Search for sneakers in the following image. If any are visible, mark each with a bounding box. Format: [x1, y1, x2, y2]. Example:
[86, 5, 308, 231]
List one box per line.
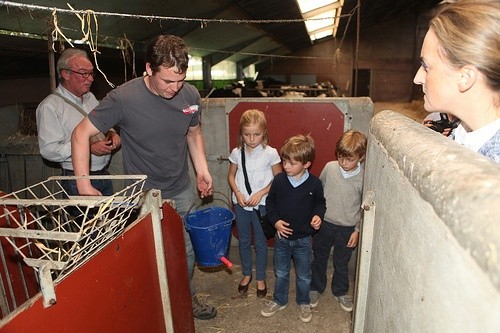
[261, 300, 288, 317]
[333, 294, 353, 312]
[298, 304, 312, 323]
[193, 305, 217, 319]
[308, 290, 321, 307]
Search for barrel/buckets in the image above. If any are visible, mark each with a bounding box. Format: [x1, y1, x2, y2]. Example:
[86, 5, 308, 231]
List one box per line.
[183, 190, 236, 268]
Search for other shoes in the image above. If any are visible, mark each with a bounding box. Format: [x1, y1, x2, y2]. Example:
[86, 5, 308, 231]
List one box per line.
[239, 274, 252, 294]
[257, 280, 266, 298]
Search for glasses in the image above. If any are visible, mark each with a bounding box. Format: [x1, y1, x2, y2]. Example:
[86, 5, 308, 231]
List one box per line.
[60, 69, 97, 78]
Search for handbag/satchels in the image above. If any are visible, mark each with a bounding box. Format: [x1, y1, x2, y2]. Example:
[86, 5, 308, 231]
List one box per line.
[259, 215, 275, 239]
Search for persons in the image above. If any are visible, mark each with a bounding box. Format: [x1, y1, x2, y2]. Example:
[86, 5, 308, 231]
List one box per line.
[413, 0, 500, 162]
[36, 49, 121, 260]
[71, 34, 217, 320]
[260, 132, 327, 322]
[228, 109, 282, 298]
[309, 129, 368, 312]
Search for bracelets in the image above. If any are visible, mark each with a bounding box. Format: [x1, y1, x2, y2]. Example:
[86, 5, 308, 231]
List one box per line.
[235, 190, 240, 194]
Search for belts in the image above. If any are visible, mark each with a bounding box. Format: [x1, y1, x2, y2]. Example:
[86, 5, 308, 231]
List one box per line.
[61, 169, 100, 175]
